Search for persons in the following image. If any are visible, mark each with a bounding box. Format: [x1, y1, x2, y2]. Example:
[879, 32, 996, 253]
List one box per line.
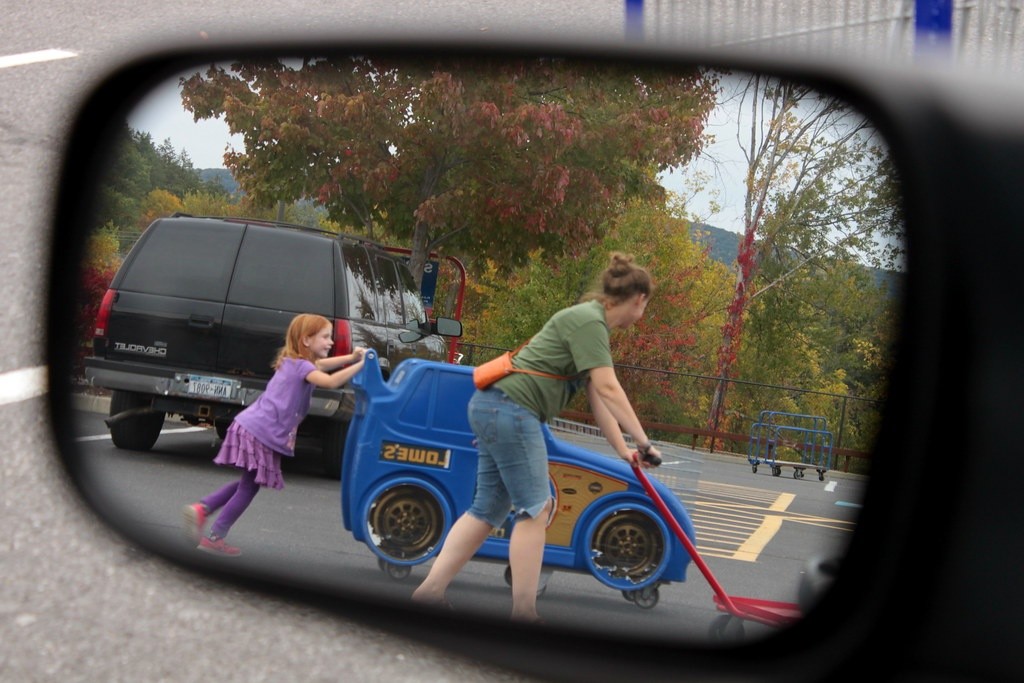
[410, 252, 662, 626]
[182, 315, 367, 557]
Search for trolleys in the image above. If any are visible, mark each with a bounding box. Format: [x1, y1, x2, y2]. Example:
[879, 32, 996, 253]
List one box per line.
[746, 410, 834, 481]
[628, 448, 810, 643]
[338, 348, 698, 612]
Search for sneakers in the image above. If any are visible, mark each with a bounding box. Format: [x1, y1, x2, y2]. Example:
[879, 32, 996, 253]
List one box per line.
[196, 536, 240, 557]
[185, 503, 206, 547]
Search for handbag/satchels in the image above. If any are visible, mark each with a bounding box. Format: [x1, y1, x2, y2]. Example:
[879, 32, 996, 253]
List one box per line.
[473, 351, 515, 389]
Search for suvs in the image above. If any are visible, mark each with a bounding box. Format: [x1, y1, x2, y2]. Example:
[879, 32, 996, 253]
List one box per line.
[82, 211, 463, 482]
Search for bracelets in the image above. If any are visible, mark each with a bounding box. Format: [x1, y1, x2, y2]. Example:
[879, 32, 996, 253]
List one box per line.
[637, 444, 651, 454]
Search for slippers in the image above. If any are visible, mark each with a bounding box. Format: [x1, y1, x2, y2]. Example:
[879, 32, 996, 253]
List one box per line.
[408, 595, 455, 611]
[511, 613, 549, 626]
